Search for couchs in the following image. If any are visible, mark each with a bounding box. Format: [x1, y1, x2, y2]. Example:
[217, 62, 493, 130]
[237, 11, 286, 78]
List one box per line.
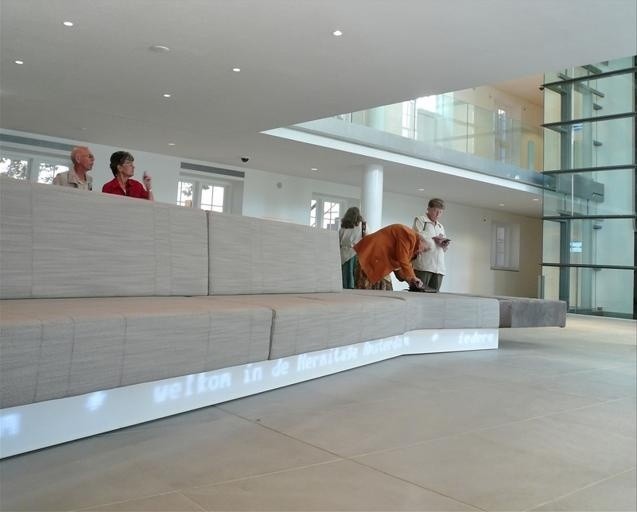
[0, 174, 499, 460]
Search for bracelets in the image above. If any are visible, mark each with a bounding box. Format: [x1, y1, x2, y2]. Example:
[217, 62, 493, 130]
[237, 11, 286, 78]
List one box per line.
[149, 188, 153, 192]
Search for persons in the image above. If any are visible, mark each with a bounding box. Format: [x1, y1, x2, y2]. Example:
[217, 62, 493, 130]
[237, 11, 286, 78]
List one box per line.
[353, 224, 434, 291]
[410, 198, 449, 293]
[339, 207, 370, 289]
[102, 151, 154, 201]
[52, 146, 94, 191]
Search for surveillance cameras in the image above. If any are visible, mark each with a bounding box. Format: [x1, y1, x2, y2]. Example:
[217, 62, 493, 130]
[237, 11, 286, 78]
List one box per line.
[241, 157, 249, 163]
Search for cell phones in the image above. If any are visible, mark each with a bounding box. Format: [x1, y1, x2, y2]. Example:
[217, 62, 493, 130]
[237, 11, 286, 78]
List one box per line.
[443, 239, 450, 242]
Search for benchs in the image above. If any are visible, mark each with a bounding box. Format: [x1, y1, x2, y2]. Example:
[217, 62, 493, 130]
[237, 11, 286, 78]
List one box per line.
[499, 296, 567, 328]
